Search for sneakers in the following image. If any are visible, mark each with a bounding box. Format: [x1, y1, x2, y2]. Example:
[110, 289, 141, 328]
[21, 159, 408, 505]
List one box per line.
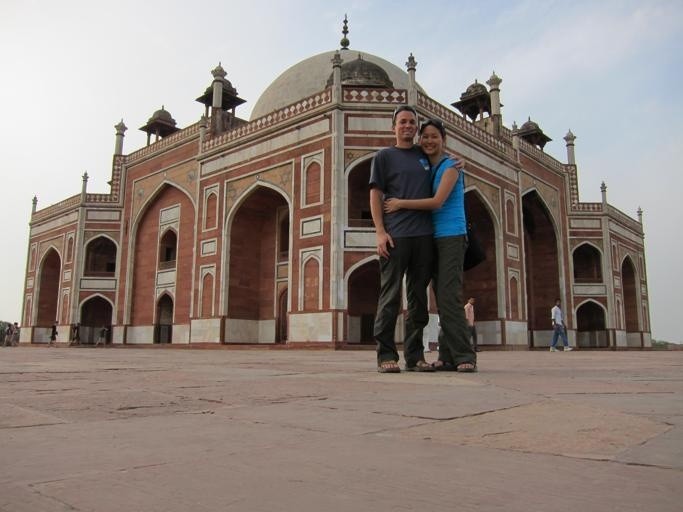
[563, 348, 572, 352]
[550, 346, 558, 352]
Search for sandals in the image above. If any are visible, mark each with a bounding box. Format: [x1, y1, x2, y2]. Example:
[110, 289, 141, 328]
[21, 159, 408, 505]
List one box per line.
[377, 361, 400, 372]
[405, 362, 475, 372]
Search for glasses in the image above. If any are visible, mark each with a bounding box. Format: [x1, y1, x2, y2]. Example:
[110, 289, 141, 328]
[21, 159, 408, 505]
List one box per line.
[393, 106, 416, 117]
[421, 119, 444, 132]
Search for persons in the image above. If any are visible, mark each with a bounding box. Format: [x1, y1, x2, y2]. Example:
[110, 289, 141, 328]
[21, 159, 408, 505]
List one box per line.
[3, 322, 109, 347]
[369, 106, 481, 373]
[549, 298, 573, 352]
